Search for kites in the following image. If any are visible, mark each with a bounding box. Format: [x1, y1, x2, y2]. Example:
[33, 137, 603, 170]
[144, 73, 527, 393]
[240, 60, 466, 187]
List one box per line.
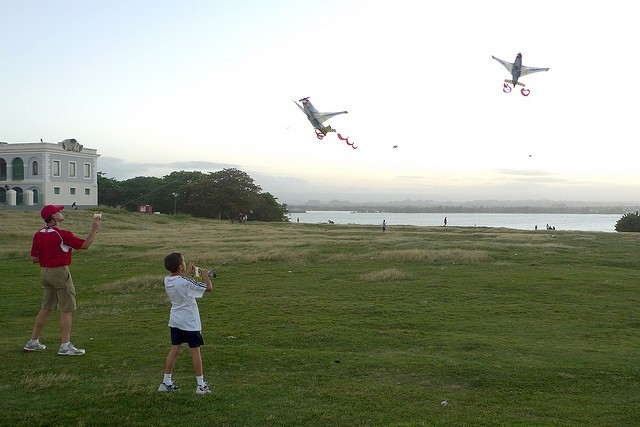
[293, 96, 358, 150]
[529, 155, 532, 157]
[393, 144, 398, 148]
[491, 52, 549, 96]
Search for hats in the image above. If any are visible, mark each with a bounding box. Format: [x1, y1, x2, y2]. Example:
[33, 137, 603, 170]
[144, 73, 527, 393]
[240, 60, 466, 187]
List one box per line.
[41, 205, 64, 219]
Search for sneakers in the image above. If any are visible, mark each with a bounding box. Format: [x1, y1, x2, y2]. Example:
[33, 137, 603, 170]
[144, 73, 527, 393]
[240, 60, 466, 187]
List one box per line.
[58, 343, 85, 355]
[158, 383, 179, 391]
[24, 341, 46, 351]
[196, 384, 211, 394]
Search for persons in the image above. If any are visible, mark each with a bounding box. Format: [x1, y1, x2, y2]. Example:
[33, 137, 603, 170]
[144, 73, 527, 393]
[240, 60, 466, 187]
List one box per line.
[382, 219, 387, 232]
[297, 217, 300, 223]
[24, 204, 102, 356]
[239, 212, 247, 224]
[158, 252, 212, 394]
[444, 217, 447, 226]
[546, 223, 555, 230]
[534, 224, 538, 229]
